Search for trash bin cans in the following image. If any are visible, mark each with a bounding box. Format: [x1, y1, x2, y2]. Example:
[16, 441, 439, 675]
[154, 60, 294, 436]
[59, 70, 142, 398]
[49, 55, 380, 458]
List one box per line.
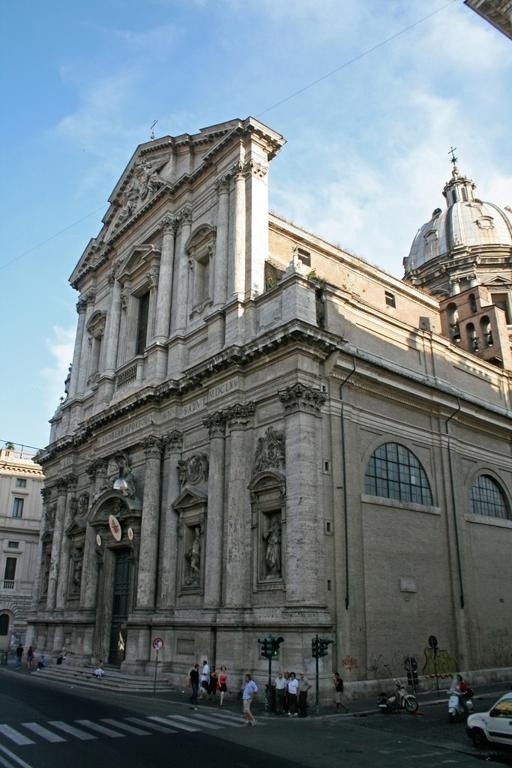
[0, 651, 8, 665]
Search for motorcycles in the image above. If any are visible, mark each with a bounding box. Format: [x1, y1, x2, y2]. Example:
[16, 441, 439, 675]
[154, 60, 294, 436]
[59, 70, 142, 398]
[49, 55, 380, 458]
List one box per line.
[446, 680, 474, 720]
[376, 678, 419, 714]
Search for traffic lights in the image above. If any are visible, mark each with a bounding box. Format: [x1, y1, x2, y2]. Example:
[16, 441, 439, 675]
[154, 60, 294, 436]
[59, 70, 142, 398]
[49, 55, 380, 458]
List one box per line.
[271, 637, 284, 657]
[263, 638, 273, 658]
[312, 638, 320, 658]
[258, 638, 265, 657]
[320, 639, 329, 657]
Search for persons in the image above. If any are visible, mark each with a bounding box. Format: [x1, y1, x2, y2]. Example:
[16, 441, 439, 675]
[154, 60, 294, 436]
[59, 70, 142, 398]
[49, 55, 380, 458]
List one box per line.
[14, 643, 24, 670]
[206, 666, 217, 707]
[332, 672, 350, 713]
[242, 674, 258, 728]
[450, 673, 468, 716]
[260, 520, 281, 577]
[188, 663, 199, 704]
[274, 671, 299, 718]
[94, 659, 105, 679]
[26, 645, 35, 670]
[216, 665, 228, 709]
[298, 672, 312, 718]
[180, 527, 201, 586]
[197, 660, 210, 702]
[37, 655, 49, 671]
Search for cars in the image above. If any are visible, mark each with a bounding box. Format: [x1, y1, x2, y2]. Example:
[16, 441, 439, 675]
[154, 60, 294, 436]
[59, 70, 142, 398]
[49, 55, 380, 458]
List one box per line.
[466, 691, 512, 749]
